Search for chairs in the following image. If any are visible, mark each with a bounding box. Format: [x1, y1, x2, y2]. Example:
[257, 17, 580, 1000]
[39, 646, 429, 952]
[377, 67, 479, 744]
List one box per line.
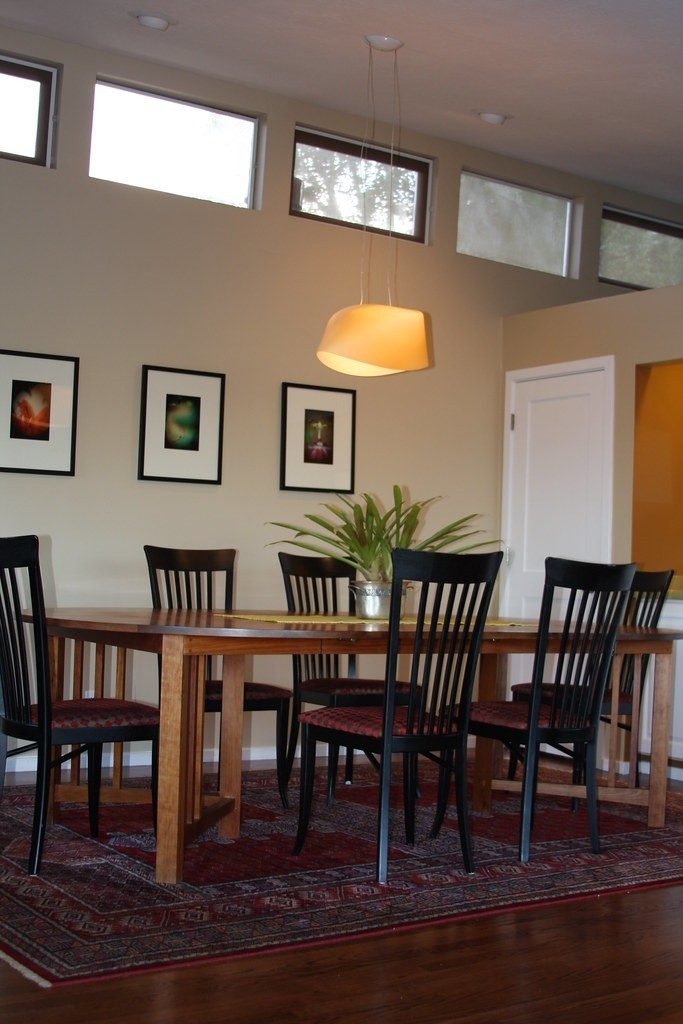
[428, 557, 637, 865]
[279, 552, 422, 806]
[0, 534, 160, 876]
[144, 544, 291, 809]
[506, 568, 675, 812]
[292, 548, 504, 885]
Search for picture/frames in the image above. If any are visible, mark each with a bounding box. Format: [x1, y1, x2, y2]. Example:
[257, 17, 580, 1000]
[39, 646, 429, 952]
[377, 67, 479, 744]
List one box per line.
[0, 349, 80, 477]
[137, 365, 225, 485]
[280, 381, 357, 494]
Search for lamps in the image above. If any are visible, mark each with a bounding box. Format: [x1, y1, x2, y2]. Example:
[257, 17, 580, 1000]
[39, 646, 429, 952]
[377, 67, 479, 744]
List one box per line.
[317, 35, 430, 377]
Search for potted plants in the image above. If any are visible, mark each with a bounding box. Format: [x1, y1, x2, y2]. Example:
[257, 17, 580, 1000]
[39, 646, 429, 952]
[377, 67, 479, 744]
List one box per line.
[264, 486, 506, 619]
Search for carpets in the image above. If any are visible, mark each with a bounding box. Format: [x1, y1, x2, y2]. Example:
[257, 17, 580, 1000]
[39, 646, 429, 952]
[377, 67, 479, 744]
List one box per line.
[0, 764, 683, 993]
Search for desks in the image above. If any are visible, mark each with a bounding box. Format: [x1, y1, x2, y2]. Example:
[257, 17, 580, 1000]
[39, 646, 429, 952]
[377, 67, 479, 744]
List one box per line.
[14, 604, 683, 885]
